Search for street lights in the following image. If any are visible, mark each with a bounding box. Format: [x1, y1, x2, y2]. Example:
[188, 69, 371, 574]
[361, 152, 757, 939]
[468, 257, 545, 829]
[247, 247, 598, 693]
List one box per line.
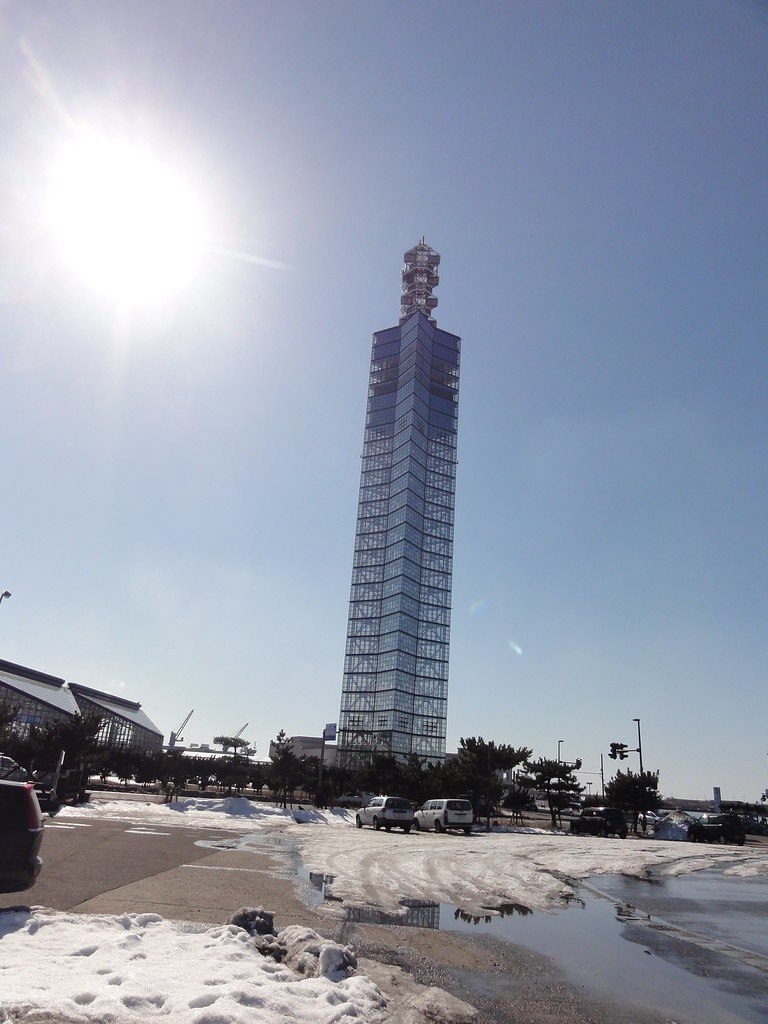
[632, 719, 643, 775]
[557, 740, 567, 811]
[587, 782, 593, 801]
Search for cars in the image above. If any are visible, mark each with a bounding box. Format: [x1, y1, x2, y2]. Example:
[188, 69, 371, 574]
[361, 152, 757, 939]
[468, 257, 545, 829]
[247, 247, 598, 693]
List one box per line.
[0, 751, 29, 783]
[0, 778, 48, 893]
[638, 809, 668, 826]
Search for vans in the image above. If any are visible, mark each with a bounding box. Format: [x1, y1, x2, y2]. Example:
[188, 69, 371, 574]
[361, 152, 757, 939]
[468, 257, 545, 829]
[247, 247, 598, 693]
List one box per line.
[414, 798, 474, 835]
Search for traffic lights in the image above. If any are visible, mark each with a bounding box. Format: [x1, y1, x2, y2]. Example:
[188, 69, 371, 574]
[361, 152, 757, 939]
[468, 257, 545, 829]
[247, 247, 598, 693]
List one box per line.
[620, 742, 628, 760]
[607, 743, 617, 759]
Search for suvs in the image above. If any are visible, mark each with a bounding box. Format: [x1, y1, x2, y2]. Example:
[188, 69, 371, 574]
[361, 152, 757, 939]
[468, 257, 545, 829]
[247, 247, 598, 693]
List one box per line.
[570, 806, 628, 840]
[688, 812, 745, 846]
[355, 796, 415, 834]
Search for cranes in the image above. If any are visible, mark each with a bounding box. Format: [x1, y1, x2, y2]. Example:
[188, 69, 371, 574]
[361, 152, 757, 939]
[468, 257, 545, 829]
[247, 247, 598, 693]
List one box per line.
[222, 722, 250, 752]
[168, 709, 195, 748]
